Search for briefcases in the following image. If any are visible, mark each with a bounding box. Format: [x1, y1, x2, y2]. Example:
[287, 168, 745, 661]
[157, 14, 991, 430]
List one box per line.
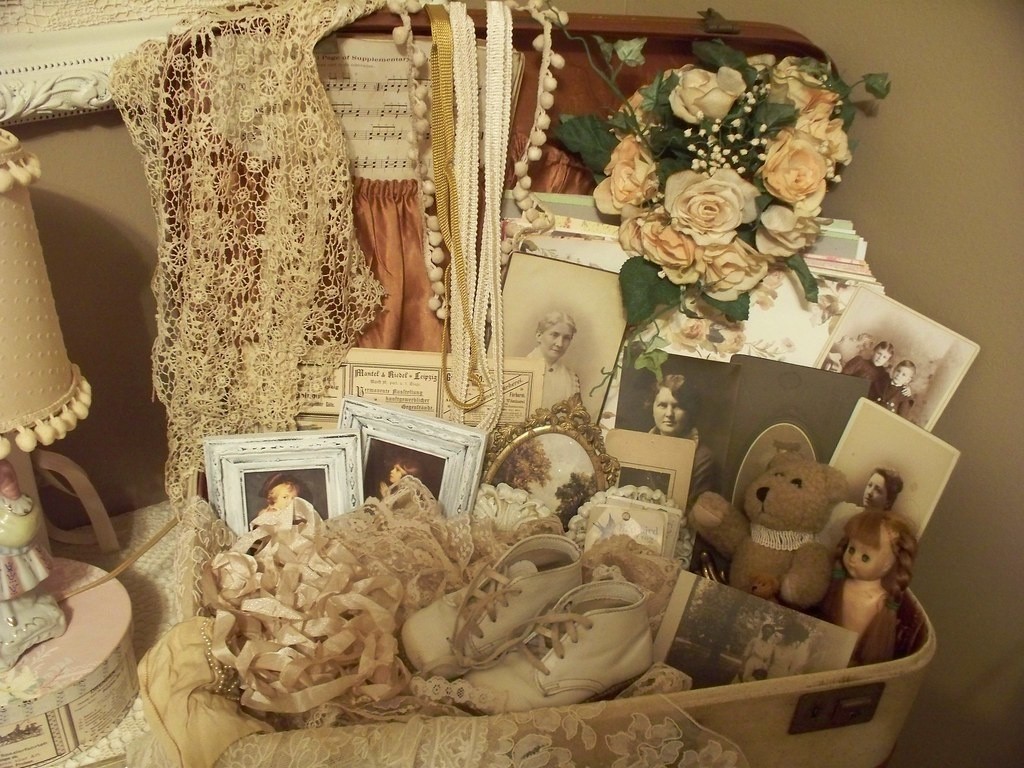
[157, 6, 938, 768]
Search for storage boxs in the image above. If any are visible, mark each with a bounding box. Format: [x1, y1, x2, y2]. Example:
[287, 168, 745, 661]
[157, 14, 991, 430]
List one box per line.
[138, 0, 938, 768]
[0, 558, 140, 768]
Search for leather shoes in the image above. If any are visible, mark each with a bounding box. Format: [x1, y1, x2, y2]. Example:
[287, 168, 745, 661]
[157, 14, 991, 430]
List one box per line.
[398, 534, 583, 679]
[462, 580, 653, 716]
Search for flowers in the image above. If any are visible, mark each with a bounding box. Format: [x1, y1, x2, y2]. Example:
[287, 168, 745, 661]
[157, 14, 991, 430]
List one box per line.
[540, 10, 896, 431]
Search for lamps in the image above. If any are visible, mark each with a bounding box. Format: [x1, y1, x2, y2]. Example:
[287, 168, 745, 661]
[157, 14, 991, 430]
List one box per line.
[0, 128, 135, 558]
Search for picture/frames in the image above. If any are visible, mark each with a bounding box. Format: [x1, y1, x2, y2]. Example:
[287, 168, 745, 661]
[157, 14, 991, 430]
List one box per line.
[485, 251, 630, 423]
[339, 392, 489, 520]
[811, 283, 981, 432]
[829, 397, 961, 543]
[202, 428, 365, 559]
[565, 483, 693, 575]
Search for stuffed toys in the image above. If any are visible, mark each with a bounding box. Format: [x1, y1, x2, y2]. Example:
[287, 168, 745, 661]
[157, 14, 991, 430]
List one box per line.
[688, 454, 849, 610]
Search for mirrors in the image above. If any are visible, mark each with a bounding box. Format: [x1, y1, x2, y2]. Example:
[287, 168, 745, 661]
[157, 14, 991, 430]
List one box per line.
[478, 392, 621, 532]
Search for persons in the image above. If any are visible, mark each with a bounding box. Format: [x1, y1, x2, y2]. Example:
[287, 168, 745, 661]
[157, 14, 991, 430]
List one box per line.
[379, 456, 424, 500]
[819, 466, 904, 549]
[824, 333, 916, 419]
[813, 507, 916, 722]
[639, 374, 721, 508]
[259, 472, 309, 507]
[731, 623, 777, 683]
[524, 312, 581, 409]
